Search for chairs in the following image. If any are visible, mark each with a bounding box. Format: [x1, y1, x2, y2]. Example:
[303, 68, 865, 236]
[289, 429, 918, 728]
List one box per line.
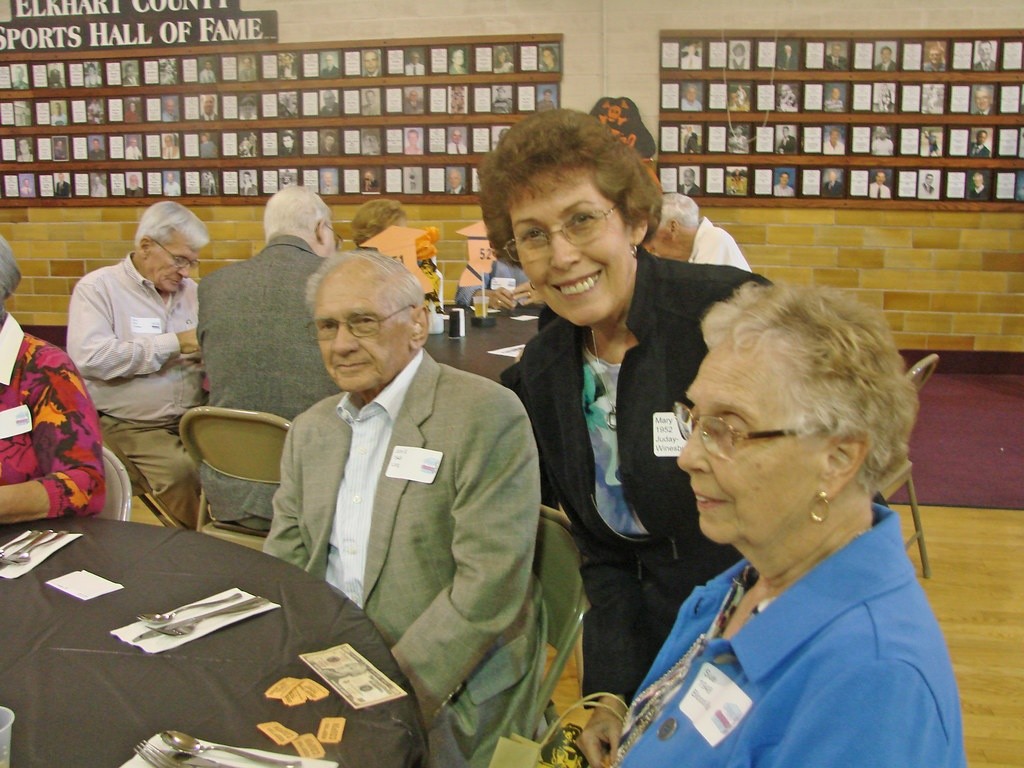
[178, 406, 292, 554]
[532, 503, 589, 728]
[877, 354, 940, 577]
[96, 445, 131, 522]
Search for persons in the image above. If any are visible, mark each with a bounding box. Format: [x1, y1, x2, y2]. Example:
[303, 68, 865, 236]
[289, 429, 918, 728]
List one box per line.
[675, 37, 996, 203]
[644, 194, 752, 272]
[478, 108, 778, 768]
[198, 184, 342, 527]
[10, 44, 559, 203]
[609, 279, 964, 768]
[455, 254, 546, 310]
[264, 242, 541, 768]
[351, 199, 405, 251]
[66, 200, 210, 530]
[0, 232, 106, 525]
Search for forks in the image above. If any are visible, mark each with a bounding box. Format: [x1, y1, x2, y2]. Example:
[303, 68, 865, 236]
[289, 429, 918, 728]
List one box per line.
[133, 739, 205, 768]
[1, 530, 70, 564]
[146, 597, 269, 637]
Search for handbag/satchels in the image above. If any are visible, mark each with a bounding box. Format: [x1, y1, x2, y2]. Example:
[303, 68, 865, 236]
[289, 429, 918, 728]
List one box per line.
[490, 693, 629, 768]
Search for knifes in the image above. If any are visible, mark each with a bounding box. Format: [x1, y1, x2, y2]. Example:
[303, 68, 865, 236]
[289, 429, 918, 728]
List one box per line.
[160, 749, 235, 768]
[0, 529, 53, 565]
[132, 596, 262, 643]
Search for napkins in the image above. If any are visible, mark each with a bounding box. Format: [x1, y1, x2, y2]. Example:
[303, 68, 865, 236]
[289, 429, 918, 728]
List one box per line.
[109, 586, 282, 653]
[0, 530, 83, 580]
[47, 570, 124, 600]
[119, 734, 339, 768]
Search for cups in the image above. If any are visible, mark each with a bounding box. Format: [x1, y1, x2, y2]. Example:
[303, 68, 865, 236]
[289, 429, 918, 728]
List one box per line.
[472, 296, 489, 318]
[0, 705, 16, 768]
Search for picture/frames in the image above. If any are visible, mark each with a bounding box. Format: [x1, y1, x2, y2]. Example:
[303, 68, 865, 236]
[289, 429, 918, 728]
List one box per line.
[655, 38, 1023, 204]
[9, 40, 564, 199]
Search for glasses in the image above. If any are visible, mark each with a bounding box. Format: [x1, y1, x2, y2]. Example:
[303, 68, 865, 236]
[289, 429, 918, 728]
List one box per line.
[318, 221, 343, 250]
[146, 235, 201, 269]
[306, 305, 415, 341]
[504, 203, 620, 263]
[673, 402, 837, 458]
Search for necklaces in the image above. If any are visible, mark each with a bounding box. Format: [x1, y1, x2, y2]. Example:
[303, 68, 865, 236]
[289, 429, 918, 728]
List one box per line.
[606, 523, 874, 768]
[590, 328, 616, 430]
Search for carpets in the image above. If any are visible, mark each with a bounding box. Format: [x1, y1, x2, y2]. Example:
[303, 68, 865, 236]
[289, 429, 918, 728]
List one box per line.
[886, 373, 1024, 511]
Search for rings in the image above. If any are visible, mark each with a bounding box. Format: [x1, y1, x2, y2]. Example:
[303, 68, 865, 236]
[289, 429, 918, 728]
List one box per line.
[527, 292, 531, 296]
[498, 300, 501, 303]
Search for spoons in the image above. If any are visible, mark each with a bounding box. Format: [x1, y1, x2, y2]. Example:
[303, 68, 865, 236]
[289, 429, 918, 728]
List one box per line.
[161, 730, 302, 768]
[0, 529, 41, 558]
[136, 593, 243, 625]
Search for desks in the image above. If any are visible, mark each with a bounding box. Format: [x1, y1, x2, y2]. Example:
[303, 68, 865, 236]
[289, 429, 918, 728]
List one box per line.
[419, 304, 542, 383]
[0, 515, 435, 768]
